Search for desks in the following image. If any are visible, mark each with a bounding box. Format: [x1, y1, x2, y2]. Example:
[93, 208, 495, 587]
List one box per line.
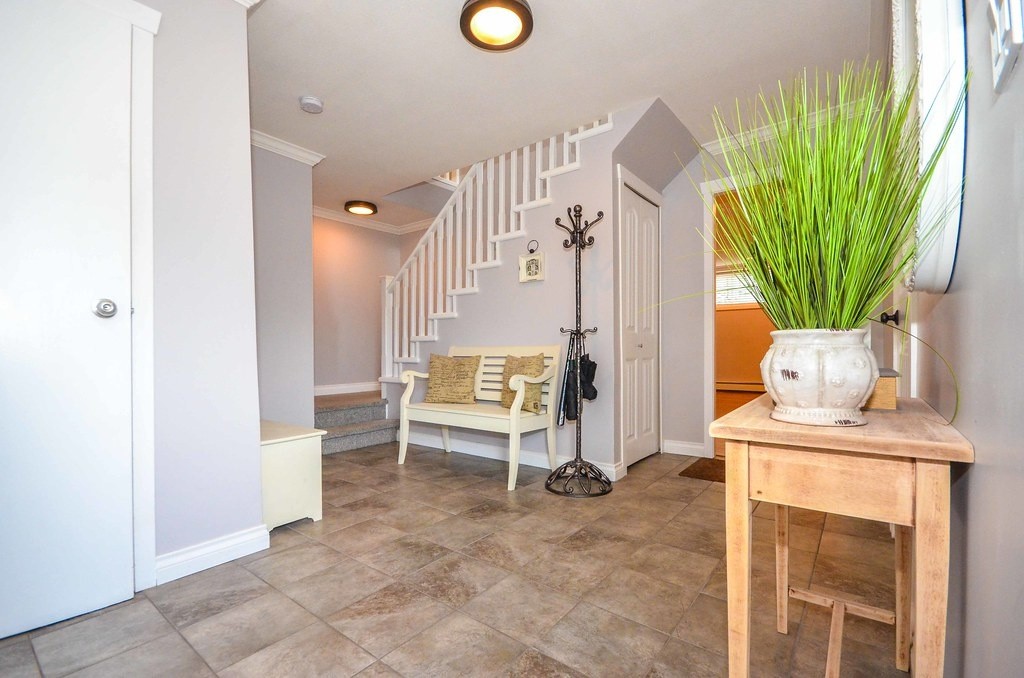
[707, 391, 975, 678]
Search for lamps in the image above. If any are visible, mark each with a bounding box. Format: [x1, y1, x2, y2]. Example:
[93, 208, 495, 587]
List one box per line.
[459, 0, 533, 51]
[343, 199, 377, 216]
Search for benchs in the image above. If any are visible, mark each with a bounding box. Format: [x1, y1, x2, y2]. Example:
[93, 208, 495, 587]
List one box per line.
[396, 345, 565, 491]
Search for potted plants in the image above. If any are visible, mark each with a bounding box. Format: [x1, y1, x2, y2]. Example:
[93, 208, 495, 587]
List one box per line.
[675, 54, 973, 429]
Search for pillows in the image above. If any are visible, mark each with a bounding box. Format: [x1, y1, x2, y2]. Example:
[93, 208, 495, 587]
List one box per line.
[501, 352, 545, 414]
[423, 353, 481, 404]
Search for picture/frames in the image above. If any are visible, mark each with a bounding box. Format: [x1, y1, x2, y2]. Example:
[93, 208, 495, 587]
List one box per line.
[518, 251, 544, 282]
[889, 0, 968, 294]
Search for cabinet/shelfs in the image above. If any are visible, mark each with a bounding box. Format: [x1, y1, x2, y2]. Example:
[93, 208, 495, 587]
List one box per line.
[259, 417, 327, 534]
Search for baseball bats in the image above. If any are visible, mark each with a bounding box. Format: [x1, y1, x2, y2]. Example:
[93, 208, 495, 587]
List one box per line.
[557, 330, 575, 426]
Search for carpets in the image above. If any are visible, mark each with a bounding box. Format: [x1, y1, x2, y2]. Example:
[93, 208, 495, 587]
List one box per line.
[678, 457, 725, 483]
